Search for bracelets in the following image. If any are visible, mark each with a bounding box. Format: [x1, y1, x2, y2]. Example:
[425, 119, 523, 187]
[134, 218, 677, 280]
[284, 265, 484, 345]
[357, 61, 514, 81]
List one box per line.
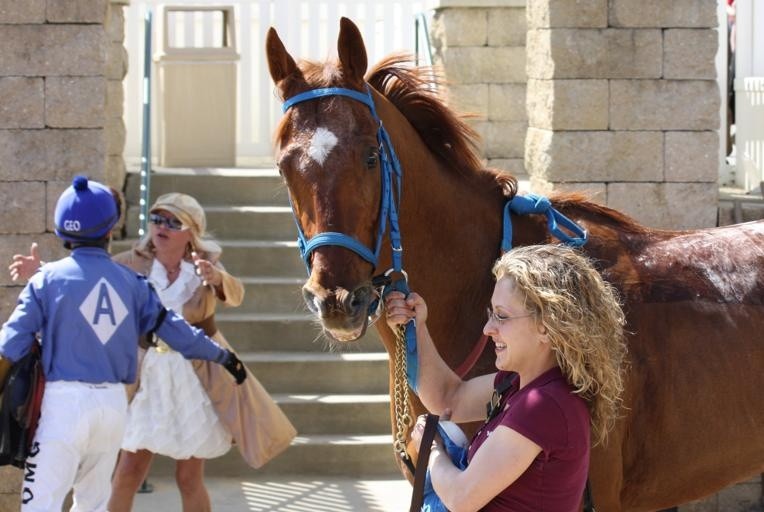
[430, 441, 442, 449]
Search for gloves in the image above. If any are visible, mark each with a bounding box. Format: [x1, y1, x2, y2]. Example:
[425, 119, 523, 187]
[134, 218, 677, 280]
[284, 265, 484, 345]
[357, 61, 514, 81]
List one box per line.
[224, 349, 246, 383]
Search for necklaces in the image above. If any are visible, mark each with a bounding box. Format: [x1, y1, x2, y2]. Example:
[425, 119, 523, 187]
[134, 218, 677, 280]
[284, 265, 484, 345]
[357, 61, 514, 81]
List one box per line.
[167, 268, 178, 273]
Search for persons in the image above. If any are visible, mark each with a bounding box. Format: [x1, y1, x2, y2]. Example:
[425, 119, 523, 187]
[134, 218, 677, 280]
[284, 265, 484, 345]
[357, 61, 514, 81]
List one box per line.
[8, 191, 298, 512]
[385, 244, 632, 511]
[1, 176, 247, 511]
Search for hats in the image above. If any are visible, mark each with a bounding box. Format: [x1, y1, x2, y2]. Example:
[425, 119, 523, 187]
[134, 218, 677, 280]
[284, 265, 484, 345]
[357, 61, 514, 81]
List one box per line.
[54, 177, 121, 242]
[148, 193, 205, 233]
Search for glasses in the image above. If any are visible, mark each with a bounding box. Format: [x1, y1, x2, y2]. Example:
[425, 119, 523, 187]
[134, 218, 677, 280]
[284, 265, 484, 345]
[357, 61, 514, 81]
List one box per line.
[486, 307, 533, 322]
[150, 214, 184, 229]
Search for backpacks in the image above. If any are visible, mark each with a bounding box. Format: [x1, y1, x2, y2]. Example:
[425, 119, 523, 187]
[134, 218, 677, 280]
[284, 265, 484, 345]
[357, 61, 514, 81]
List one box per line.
[0, 340, 45, 467]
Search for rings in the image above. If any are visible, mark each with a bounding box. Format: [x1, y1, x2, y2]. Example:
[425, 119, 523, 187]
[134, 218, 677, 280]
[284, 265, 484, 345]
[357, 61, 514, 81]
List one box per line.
[388, 311, 391, 316]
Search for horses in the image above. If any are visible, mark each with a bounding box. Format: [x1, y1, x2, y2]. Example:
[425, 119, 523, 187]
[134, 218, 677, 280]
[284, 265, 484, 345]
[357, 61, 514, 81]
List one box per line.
[262, 15, 764, 512]
